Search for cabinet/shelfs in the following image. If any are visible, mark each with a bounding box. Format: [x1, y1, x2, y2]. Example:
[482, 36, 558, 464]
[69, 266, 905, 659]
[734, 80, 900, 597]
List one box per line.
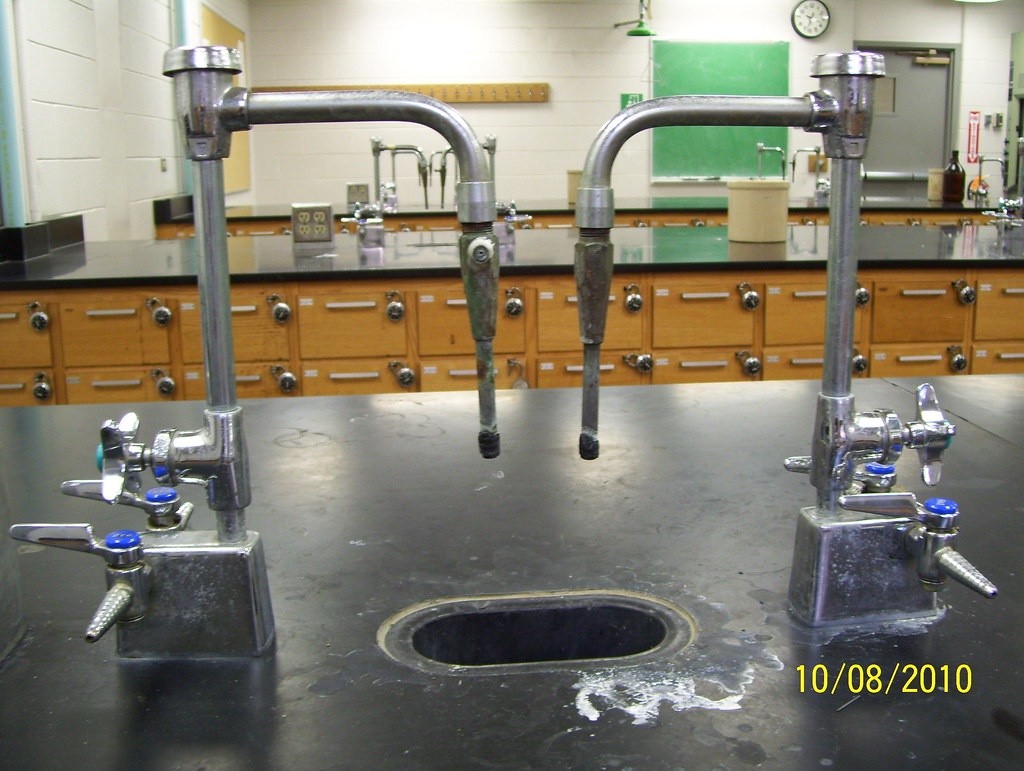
[1, 268, 1022, 404]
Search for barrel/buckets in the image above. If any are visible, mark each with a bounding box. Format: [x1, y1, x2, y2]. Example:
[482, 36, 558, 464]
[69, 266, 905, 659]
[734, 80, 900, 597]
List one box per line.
[726, 178, 791, 245]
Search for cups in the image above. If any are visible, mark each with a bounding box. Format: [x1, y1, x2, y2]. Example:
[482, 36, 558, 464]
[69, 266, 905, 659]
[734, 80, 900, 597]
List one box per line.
[928, 169, 943, 201]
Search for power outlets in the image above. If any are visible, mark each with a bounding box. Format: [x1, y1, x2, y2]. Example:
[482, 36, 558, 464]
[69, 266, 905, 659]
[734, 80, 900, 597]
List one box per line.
[346, 183, 369, 204]
[292, 204, 334, 244]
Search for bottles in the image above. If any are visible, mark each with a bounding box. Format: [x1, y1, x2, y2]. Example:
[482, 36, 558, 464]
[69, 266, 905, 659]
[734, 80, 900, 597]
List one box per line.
[942, 151, 965, 203]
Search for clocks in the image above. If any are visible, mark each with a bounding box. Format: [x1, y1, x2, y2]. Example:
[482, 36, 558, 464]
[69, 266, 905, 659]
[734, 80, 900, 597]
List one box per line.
[791, 0, 830, 39]
